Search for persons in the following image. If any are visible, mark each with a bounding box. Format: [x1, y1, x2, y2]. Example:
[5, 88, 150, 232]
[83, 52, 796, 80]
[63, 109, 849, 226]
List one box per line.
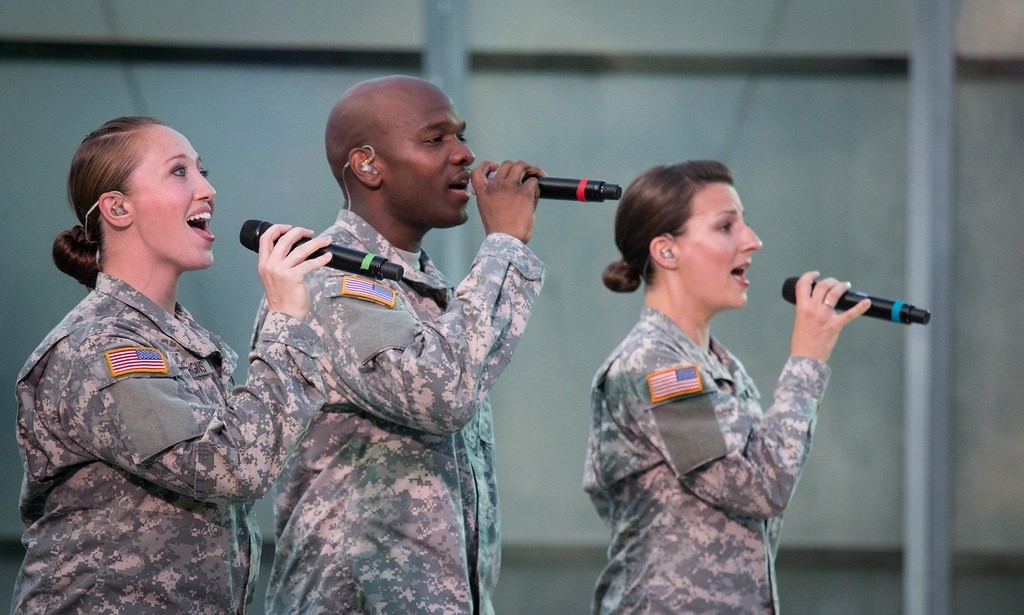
[583, 159, 871, 615]
[251, 75, 546, 615]
[12, 116, 332, 615]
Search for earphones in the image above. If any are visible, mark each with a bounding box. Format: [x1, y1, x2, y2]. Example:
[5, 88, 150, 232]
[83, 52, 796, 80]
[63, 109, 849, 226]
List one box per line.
[660, 247, 674, 258]
[361, 159, 377, 175]
[112, 199, 126, 215]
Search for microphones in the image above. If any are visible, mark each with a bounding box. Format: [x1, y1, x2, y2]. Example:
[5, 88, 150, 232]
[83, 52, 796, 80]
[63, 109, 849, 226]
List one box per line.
[239, 220, 404, 282]
[469, 172, 622, 201]
[782, 278, 932, 325]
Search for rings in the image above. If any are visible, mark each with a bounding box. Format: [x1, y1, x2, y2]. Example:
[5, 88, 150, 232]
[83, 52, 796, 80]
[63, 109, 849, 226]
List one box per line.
[824, 300, 834, 308]
